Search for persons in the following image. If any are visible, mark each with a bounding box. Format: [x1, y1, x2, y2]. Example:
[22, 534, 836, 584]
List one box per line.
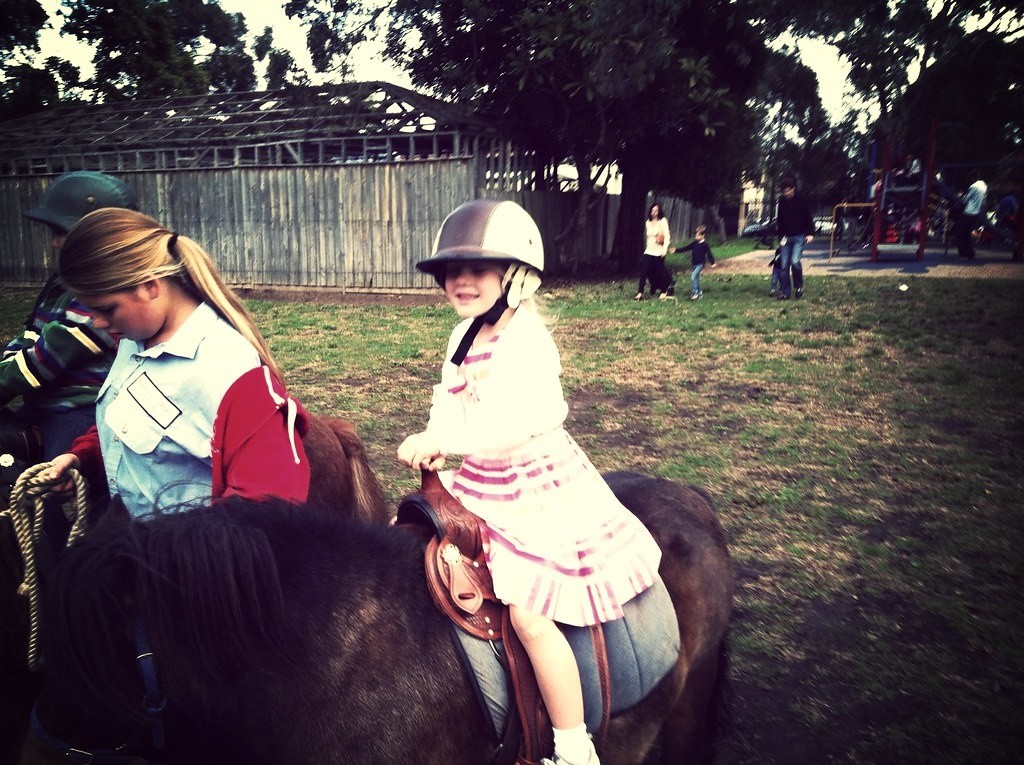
[945, 170, 990, 263]
[668, 224, 717, 301]
[398, 197, 662, 765]
[895, 150, 924, 216]
[994, 190, 1018, 246]
[634, 203, 673, 300]
[34, 207, 314, 531]
[0, 171, 142, 540]
[769, 178, 816, 299]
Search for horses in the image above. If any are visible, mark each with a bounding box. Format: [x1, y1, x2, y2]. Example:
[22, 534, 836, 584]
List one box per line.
[1, 416, 734, 765]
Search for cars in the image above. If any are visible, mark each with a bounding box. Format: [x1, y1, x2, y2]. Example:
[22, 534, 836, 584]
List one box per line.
[741, 217, 779, 237]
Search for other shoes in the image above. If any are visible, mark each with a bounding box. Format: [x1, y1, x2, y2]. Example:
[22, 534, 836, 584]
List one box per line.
[666, 287, 675, 299]
[698, 295, 704, 300]
[795, 287, 803, 300]
[953, 253, 969, 262]
[645, 294, 655, 300]
[634, 292, 643, 301]
[768, 289, 775, 297]
[691, 294, 698, 299]
[777, 293, 790, 300]
[659, 291, 667, 300]
[541, 733, 600, 765]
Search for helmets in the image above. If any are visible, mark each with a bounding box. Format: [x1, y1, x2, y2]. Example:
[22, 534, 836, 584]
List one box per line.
[416, 200, 544, 273]
[22, 170, 138, 232]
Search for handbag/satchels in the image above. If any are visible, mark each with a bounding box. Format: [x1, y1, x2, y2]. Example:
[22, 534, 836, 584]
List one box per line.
[655, 231, 663, 244]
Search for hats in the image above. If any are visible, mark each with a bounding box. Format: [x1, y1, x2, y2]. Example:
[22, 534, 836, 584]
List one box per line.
[779, 178, 795, 192]
